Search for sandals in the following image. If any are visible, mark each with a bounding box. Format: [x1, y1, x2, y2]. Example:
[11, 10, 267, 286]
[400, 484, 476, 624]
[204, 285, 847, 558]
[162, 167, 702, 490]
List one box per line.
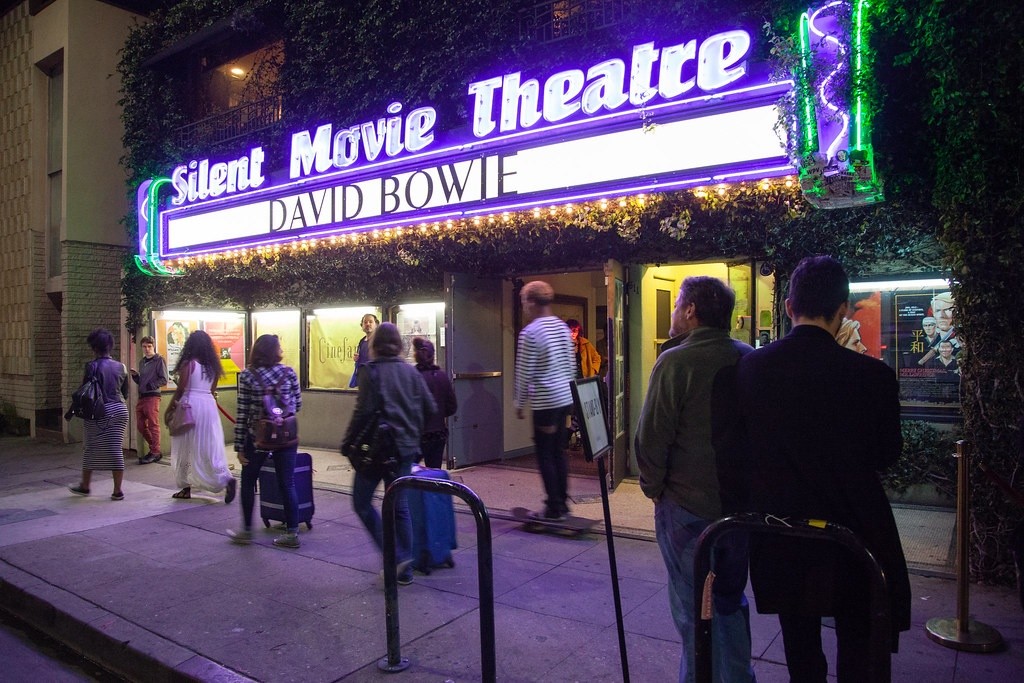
[172, 487, 191, 498]
[225, 478, 236, 503]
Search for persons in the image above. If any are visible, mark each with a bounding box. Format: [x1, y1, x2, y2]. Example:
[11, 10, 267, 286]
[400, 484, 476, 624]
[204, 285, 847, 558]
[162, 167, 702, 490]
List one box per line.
[225, 335, 301, 547]
[835, 319, 866, 354]
[711, 254, 912, 683]
[340, 322, 437, 585]
[63, 328, 129, 499]
[636, 277, 759, 683]
[350, 314, 380, 388]
[133, 336, 166, 460]
[411, 337, 457, 468]
[514, 281, 575, 522]
[566, 319, 601, 450]
[161, 331, 236, 502]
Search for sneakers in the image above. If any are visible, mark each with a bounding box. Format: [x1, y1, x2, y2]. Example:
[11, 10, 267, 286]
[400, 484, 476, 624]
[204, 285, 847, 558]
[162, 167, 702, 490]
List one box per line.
[111, 492, 124, 500]
[68, 484, 90, 496]
[224, 528, 253, 544]
[272, 534, 302, 548]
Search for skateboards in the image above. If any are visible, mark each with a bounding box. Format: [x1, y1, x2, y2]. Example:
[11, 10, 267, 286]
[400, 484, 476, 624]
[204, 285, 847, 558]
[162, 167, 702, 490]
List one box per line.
[513, 506, 604, 539]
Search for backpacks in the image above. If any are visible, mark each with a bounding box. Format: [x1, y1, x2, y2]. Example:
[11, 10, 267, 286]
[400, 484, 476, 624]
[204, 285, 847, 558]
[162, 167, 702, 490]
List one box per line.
[249, 367, 299, 450]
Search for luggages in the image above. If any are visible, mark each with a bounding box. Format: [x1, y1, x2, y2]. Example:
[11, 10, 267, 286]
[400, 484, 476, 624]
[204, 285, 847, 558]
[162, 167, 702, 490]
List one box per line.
[407, 463, 458, 575]
[259, 453, 315, 530]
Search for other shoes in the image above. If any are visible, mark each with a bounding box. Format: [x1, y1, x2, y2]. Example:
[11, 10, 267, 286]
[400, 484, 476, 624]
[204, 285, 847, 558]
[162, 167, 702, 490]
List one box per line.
[527, 511, 567, 521]
[138, 451, 162, 464]
[379, 559, 415, 586]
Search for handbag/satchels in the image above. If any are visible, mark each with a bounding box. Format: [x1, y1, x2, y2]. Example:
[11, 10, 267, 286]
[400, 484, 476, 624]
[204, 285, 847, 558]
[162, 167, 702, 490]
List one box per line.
[165, 403, 195, 436]
[349, 362, 359, 388]
[71, 362, 106, 420]
[348, 422, 404, 481]
